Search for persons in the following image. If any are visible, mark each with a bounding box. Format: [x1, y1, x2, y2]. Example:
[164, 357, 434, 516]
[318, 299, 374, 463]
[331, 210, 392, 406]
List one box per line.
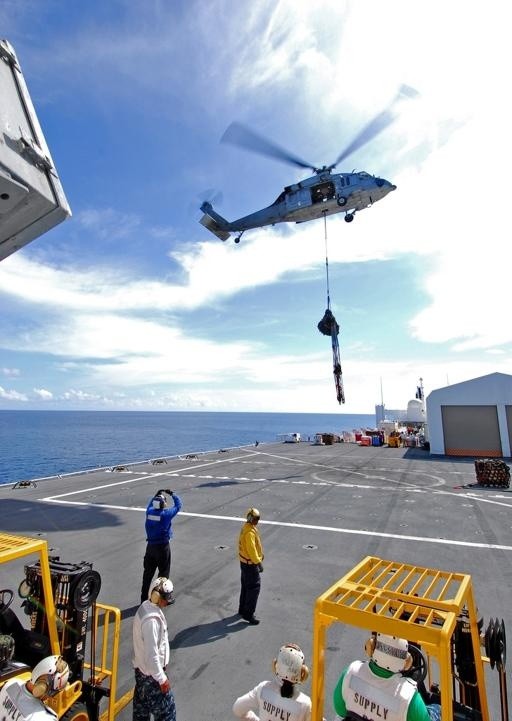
[0, 634, 30, 677]
[1, 655, 70, 721]
[333, 632, 442, 720]
[398, 425, 418, 448]
[140, 489, 184, 604]
[130, 577, 179, 720]
[238, 507, 263, 624]
[233, 643, 312, 721]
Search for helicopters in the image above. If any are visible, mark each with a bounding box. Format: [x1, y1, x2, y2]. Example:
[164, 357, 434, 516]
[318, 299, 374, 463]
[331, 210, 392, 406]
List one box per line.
[199, 85, 421, 244]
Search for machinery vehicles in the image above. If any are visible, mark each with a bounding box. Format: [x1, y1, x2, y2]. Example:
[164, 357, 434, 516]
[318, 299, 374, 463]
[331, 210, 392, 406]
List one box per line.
[1, 532, 137, 721]
[386, 429, 400, 449]
[310, 552, 508, 721]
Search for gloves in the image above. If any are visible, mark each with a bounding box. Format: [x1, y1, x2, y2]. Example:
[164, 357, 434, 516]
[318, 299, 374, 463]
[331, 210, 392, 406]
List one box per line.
[165, 490, 174, 496]
[160, 678, 171, 694]
[155, 490, 164, 495]
[259, 564, 263, 573]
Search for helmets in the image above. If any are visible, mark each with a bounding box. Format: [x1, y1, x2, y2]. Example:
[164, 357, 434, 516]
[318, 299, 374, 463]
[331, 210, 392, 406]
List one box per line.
[149, 577, 175, 607]
[152, 494, 168, 509]
[30, 655, 70, 699]
[272, 643, 309, 684]
[364, 633, 413, 673]
[246, 508, 260, 523]
